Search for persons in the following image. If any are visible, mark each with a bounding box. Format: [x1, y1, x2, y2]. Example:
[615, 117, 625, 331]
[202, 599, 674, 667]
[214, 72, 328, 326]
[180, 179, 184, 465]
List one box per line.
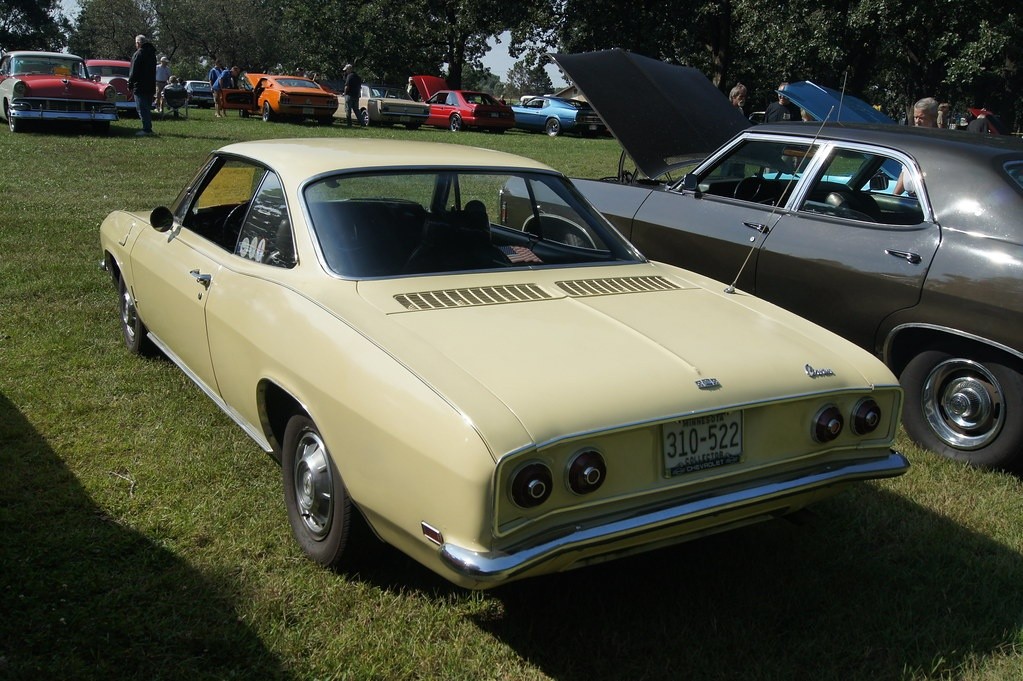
[499, 95, 506, 105]
[128, 34, 157, 135]
[156, 56, 187, 116]
[296, 67, 316, 81]
[343, 64, 366, 128]
[209, 59, 250, 118]
[405, 77, 418, 101]
[893, 97, 939, 196]
[967, 114, 988, 134]
[937, 104, 949, 129]
[760, 82, 816, 173]
[721, 83, 747, 177]
[956, 108, 973, 131]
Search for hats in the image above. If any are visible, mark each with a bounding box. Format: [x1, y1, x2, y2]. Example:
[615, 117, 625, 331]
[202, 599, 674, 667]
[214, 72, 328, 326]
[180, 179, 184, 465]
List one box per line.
[342, 63, 352, 72]
[161, 56, 171, 63]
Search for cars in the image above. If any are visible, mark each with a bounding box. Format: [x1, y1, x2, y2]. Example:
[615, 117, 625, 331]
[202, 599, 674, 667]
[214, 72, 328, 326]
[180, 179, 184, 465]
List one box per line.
[216, 74, 338, 125]
[79, 59, 139, 118]
[411, 76, 516, 135]
[100, 137, 912, 592]
[0, 50, 119, 133]
[333, 83, 430, 128]
[511, 96, 607, 137]
[180, 80, 215, 109]
[499, 50, 1023, 465]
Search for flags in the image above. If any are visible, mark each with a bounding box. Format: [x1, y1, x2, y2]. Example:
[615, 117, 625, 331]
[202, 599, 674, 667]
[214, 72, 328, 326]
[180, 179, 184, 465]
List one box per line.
[500, 246, 543, 262]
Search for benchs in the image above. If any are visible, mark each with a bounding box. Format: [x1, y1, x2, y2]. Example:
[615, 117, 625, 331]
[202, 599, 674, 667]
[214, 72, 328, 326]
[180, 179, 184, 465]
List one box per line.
[309, 202, 434, 277]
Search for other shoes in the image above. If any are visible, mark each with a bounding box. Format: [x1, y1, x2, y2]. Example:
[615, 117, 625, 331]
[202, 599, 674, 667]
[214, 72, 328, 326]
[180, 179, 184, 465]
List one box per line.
[214, 111, 221, 117]
[134, 130, 153, 136]
[361, 123, 367, 128]
[346, 124, 352, 128]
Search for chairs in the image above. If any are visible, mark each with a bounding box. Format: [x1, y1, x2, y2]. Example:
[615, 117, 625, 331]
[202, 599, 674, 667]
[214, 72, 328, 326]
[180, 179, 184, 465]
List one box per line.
[295, 81, 306, 86]
[804, 181, 885, 225]
[161, 87, 189, 120]
[402, 208, 514, 274]
